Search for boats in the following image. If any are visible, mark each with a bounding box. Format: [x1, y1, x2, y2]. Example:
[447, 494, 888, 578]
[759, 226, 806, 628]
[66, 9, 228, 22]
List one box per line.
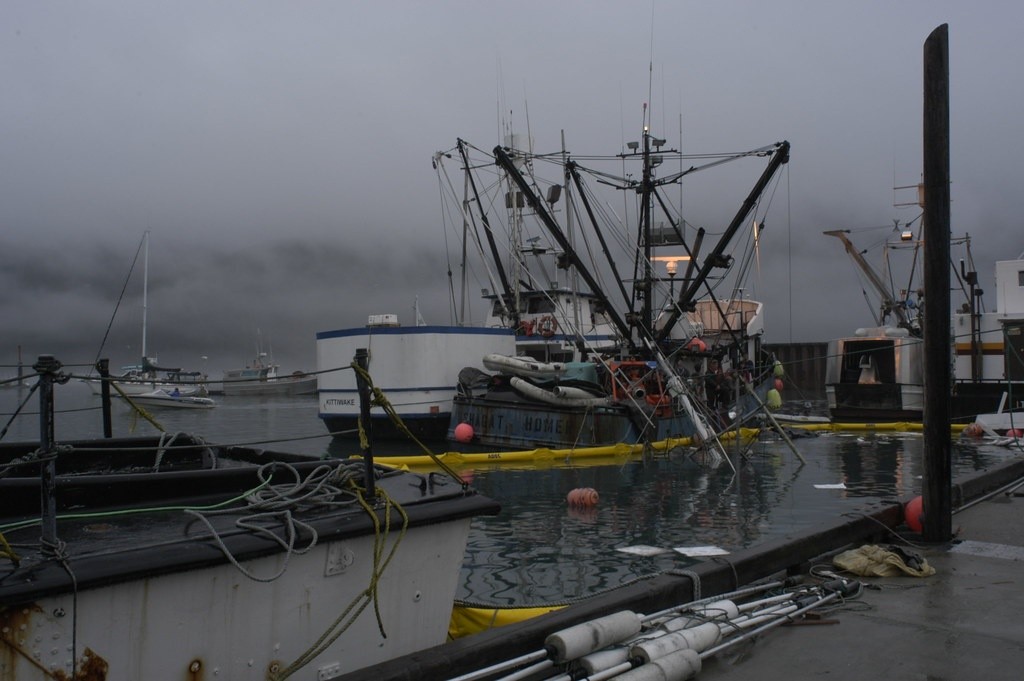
[222, 328, 317, 396]
[314, 60, 1024, 463]
[114, 388, 218, 409]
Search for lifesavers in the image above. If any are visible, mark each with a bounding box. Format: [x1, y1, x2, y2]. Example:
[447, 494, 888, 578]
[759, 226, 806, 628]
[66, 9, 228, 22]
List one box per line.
[538, 315, 557, 337]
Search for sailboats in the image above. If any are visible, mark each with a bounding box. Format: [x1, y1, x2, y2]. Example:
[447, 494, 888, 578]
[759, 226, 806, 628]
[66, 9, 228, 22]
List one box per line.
[74, 228, 210, 397]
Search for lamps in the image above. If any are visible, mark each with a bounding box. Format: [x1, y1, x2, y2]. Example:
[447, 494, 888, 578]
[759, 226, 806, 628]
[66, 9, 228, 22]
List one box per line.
[685, 299, 698, 314]
[481, 288, 489, 296]
[594, 300, 606, 315]
[902, 230, 914, 240]
[550, 282, 558, 290]
[556, 252, 571, 271]
[547, 183, 561, 209]
[652, 140, 666, 151]
[704, 254, 733, 269]
[627, 142, 640, 154]
[624, 311, 640, 325]
